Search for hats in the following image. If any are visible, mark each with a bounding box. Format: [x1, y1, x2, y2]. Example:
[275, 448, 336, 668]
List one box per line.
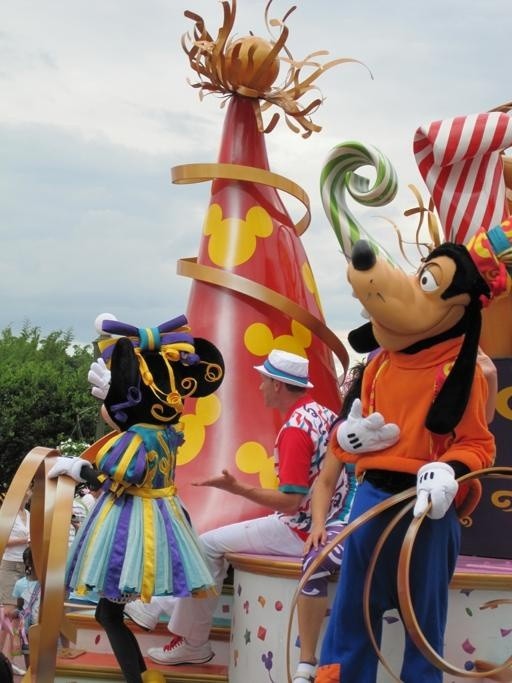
[253, 349, 314, 388]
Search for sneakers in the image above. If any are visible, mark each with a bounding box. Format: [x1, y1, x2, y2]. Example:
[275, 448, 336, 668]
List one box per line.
[147, 635, 215, 665]
[122, 600, 159, 631]
[11, 663, 26, 675]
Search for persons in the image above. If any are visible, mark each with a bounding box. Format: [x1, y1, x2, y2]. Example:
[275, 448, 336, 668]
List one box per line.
[0, 484, 103, 683]
[123, 350, 349, 667]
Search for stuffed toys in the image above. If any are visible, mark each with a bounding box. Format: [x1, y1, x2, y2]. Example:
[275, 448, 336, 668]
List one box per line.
[50, 313, 224, 683]
[313, 216, 512, 683]
